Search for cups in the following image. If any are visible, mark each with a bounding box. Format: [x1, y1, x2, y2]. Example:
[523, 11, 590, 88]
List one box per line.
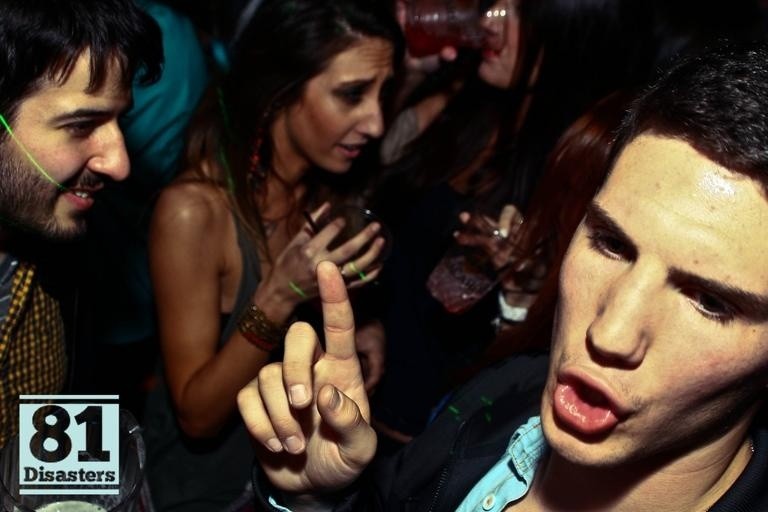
[405, 0, 507, 58]
[329, 204, 393, 277]
[0, 406, 153, 512]
[426, 213, 526, 313]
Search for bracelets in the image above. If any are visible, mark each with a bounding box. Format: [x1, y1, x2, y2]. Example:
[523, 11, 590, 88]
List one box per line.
[236, 301, 282, 352]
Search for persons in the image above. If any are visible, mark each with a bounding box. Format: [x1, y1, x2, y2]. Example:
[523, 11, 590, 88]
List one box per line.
[235, 37, 767, 512]
[0, 0, 166, 450]
[462, 88, 642, 371]
[367, 2, 624, 468]
[150, 1, 403, 438]
